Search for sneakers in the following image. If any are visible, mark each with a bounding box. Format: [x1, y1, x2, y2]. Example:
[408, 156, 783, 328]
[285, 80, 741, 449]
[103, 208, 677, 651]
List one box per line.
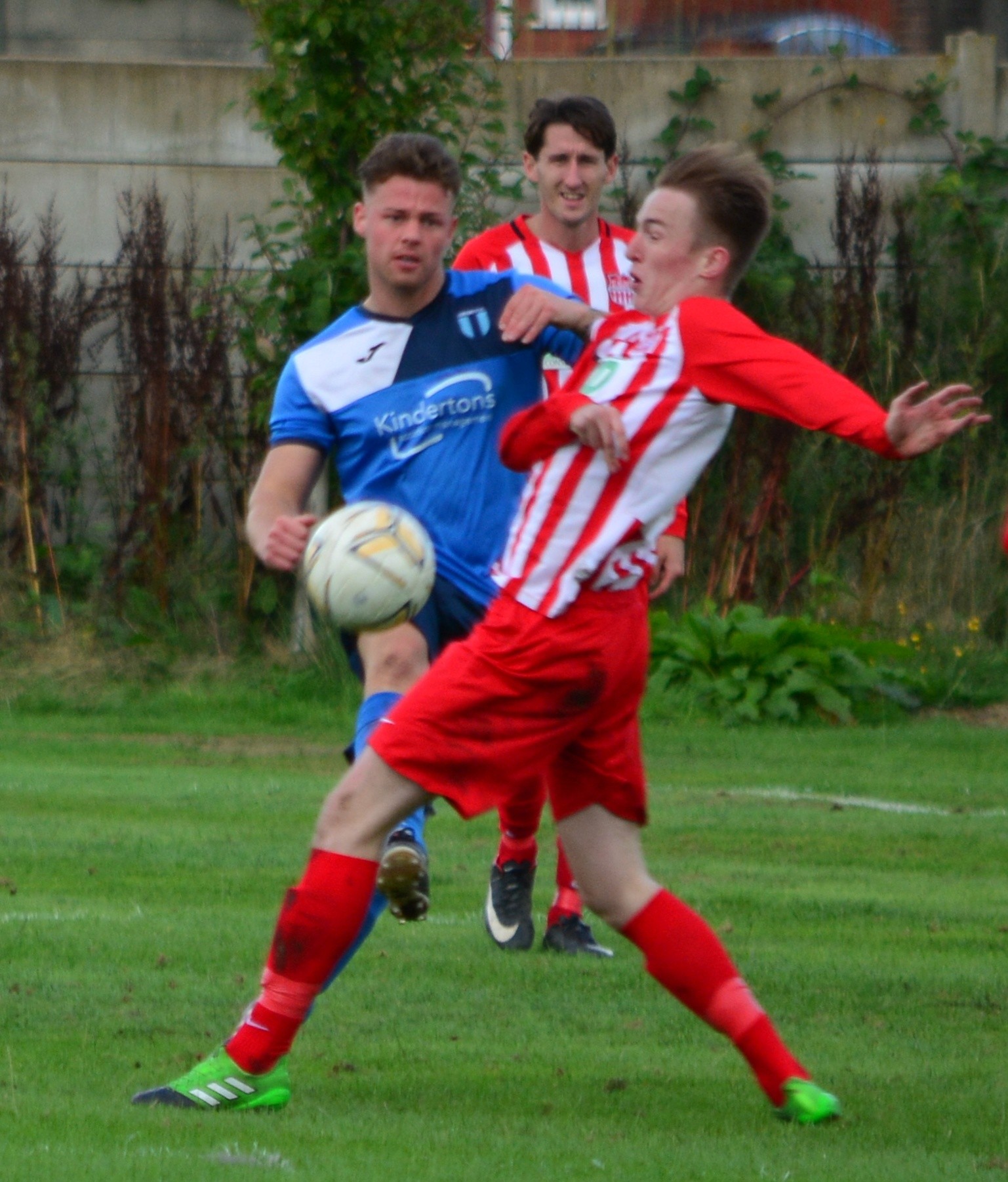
[780, 1079, 841, 1124]
[540, 915, 611, 952]
[130, 1047, 290, 1113]
[485, 855, 535, 949]
[379, 827, 432, 923]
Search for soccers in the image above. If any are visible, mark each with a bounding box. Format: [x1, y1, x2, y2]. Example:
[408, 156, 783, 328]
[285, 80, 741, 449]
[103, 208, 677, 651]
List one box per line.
[303, 501, 437, 634]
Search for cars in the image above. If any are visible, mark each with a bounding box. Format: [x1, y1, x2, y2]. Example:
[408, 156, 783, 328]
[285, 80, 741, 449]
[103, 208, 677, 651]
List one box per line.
[741, 14, 902, 57]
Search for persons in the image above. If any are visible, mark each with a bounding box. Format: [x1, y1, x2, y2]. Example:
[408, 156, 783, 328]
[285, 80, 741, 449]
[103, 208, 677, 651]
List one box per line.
[449, 93, 687, 955]
[244, 136, 612, 1029]
[132, 143, 991, 1113]
